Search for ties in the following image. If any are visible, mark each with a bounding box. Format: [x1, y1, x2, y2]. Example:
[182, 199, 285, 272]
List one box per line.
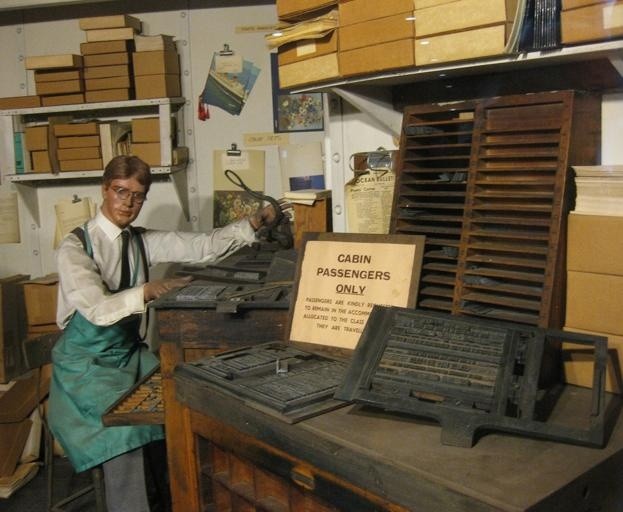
[118, 230, 130, 290]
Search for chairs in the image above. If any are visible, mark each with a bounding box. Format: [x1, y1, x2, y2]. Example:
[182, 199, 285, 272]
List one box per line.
[36, 340, 106, 512]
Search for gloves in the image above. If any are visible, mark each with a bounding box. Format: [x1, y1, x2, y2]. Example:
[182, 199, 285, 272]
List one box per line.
[249, 198, 294, 232]
[144, 276, 192, 302]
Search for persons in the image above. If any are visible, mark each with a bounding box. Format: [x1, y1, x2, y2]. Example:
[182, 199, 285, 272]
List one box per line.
[47, 155, 294, 512]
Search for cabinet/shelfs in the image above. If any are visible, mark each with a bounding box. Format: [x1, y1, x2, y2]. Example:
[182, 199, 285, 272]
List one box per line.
[0, 97, 190, 228]
[289, 37, 623, 140]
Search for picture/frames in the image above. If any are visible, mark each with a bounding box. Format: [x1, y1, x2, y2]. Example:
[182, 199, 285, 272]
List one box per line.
[284, 232, 424, 364]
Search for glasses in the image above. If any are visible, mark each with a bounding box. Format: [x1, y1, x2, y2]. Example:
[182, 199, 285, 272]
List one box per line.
[109, 185, 148, 204]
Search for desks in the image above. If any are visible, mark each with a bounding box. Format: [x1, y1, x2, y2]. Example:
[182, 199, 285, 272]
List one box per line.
[149, 248, 297, 512]
[173, 367, 623, 512]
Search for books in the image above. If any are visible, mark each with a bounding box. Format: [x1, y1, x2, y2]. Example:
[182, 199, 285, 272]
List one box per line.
[570, 165, 623, 216]
[287, 199, 315, 205]
[54, 198, 95, 249]
[135, 34, 176, 51]
[285, 189, 331, 200]
[16, 273, 59, 285]
[506, 0, 562, 53]
[100, 121, 132, 169]
